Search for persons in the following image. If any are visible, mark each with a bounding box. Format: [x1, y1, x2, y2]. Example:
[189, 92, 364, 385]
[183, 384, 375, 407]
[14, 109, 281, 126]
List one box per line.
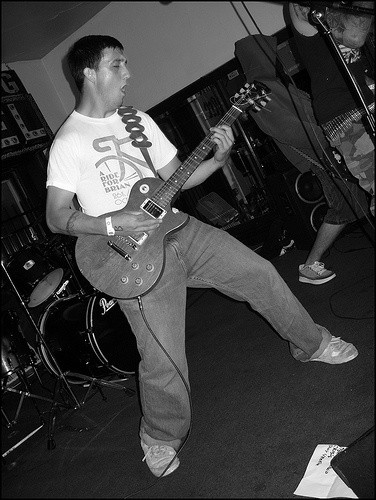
[284, 0, 376, 285]
[44, 35, 360, 478]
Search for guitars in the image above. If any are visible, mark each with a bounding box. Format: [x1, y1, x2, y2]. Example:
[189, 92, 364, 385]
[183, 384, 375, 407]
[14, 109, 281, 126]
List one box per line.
[323, 145, 359, 184]
[75, 80, 272, 300]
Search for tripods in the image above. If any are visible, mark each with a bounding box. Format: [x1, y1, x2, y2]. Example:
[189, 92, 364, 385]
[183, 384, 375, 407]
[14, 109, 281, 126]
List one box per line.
[0, 260, 136, 459]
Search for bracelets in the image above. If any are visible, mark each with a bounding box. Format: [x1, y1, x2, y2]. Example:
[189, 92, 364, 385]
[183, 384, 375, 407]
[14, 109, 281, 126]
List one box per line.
[68, 209, 83, 236]
[105, 216, 115, 237]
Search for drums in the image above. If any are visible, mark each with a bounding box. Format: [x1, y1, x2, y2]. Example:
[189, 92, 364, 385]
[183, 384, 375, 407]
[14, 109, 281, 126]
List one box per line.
[1, 242, 64, 308]
[38, 293, 141, 384]
[0, 330, 42, 393]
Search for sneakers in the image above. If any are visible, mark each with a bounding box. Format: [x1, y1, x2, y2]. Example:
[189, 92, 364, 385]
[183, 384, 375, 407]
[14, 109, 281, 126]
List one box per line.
[140, 439, 182, 478]
[297, 261, 337, 285]
[306, 335, 359, 365]
